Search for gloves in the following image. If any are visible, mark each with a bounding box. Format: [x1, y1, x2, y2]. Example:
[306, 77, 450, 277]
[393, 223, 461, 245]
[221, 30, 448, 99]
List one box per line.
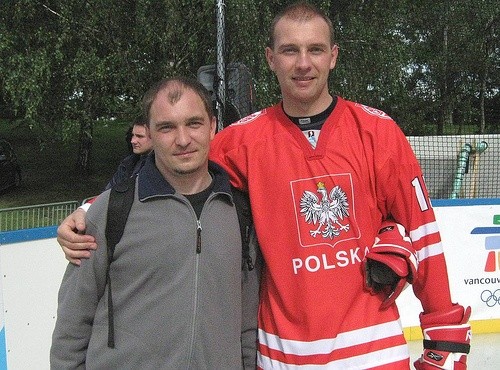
[413, 303, 472, 370]
[363, 219, 419, 311]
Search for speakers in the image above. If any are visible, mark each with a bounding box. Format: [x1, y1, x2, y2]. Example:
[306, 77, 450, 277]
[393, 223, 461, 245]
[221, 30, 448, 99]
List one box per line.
[197, 63, 254, 135]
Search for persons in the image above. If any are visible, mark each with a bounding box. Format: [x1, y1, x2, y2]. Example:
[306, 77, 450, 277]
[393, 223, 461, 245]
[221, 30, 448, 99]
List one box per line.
[57, 2, 473, 370]
[106, 115, 153, 190]
[49, 75, 261, 370]
